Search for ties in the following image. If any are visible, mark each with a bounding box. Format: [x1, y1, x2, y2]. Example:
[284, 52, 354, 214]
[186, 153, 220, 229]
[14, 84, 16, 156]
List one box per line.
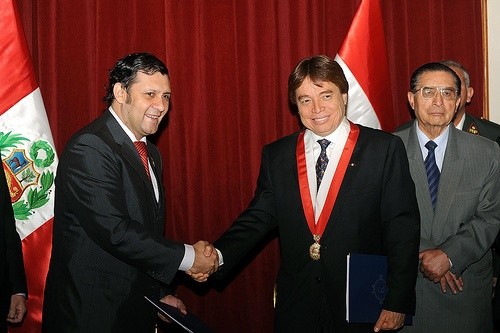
[133, 142, 150, 176]
[423, 140, 440, 210]
[314, 138, 329, 191]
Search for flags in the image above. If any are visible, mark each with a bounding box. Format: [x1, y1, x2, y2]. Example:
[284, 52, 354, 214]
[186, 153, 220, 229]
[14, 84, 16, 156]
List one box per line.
[0, 0, 59, 333]
[334, 0, 393, 133]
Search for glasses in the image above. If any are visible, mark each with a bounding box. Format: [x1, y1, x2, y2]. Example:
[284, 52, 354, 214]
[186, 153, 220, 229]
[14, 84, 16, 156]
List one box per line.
[414, 86, 458, 101]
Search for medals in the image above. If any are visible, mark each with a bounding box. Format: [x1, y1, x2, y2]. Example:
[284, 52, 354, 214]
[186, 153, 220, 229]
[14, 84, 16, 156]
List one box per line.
[310, 243, 320, 260]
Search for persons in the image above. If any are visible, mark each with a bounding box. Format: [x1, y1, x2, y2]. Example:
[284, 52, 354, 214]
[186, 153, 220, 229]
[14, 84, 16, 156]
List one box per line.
[185, 56, 421, 333]
[391, 60, 500, 333]
[41, 52, 218, 333]
[0, 151, 28, 333]
